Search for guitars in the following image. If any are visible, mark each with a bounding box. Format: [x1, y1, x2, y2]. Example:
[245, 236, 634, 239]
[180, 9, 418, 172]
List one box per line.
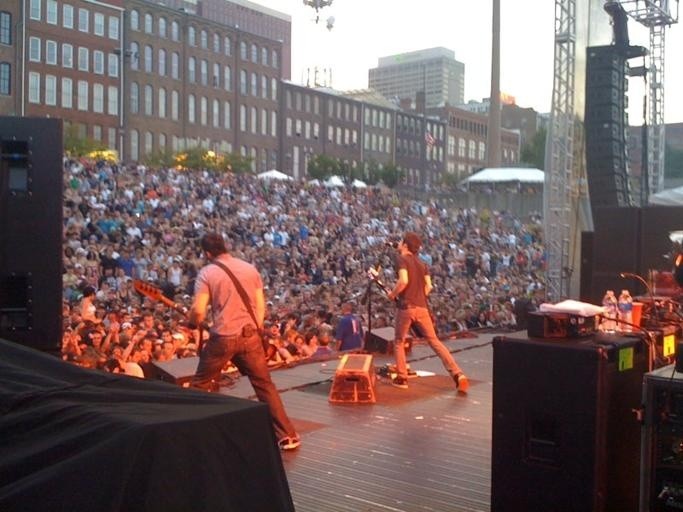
[366, 267, 437, 340]
[133, 278, 277, 377]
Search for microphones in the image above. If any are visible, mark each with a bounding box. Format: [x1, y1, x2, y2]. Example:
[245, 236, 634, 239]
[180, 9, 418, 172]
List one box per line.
[617, 271, 662, 328]
[384, 240, 400, 250]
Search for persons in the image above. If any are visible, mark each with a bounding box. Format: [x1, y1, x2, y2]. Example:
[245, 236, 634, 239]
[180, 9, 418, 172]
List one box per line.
[386, 232, 469, 393]
[186, 232, 302, 451]
[60, 148, 544, 381]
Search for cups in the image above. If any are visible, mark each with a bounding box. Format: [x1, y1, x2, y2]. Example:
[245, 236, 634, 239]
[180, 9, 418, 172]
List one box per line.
[633, 302, 643, 332]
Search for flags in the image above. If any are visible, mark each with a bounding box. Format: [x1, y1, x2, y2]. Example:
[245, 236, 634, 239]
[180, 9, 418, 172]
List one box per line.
[424, 131, 435, 146]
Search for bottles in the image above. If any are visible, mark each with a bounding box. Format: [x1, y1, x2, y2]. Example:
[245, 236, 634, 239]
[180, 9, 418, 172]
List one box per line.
[601, 290, 617, 334]
[618, 289, 634, 333]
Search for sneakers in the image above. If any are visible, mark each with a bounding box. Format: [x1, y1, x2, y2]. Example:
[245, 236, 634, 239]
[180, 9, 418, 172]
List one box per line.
[393, 377, 409, 388]
[280, 433, 301, 449]
[454, 372, 468, 392]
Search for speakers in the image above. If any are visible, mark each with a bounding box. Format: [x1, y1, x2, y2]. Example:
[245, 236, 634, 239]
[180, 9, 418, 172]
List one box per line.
[364, 326, 412, 355]
[150, 356, 200, 388]
